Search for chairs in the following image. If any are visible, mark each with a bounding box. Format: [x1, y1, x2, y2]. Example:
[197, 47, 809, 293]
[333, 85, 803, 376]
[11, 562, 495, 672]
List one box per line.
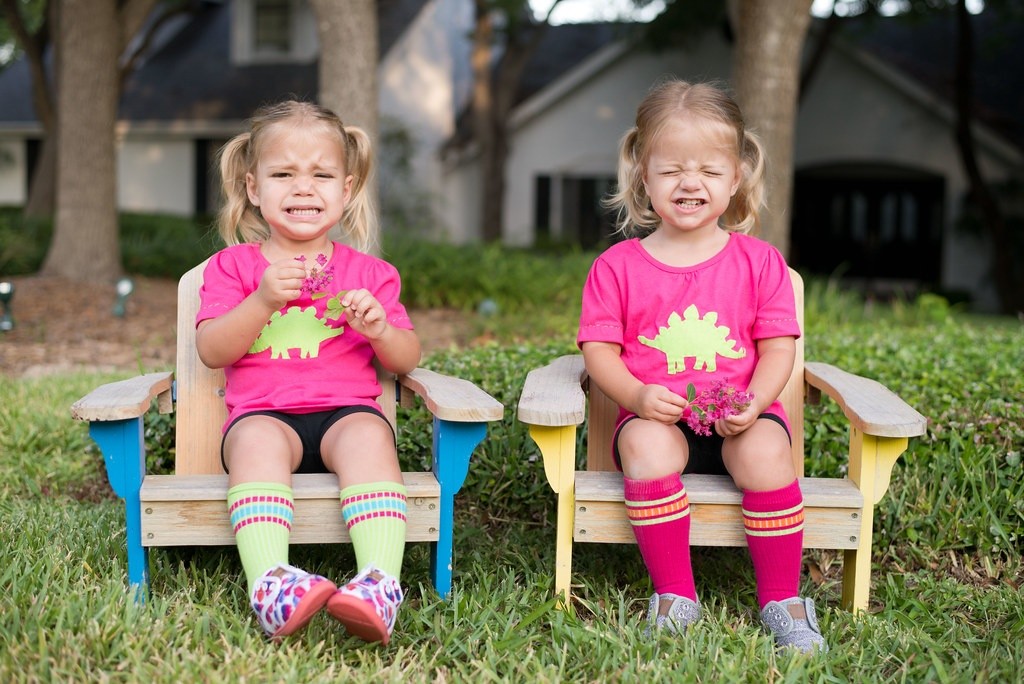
[518, 265, 927, 619]
[71, 254, 504, 604]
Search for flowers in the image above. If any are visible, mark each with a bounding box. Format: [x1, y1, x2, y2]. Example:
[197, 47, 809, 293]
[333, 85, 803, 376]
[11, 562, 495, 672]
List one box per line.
[680, 375, 755, 437]
[294, 254, 346, 321]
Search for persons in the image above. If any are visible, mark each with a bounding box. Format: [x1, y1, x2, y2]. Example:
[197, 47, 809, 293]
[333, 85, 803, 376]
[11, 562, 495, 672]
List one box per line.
[195, 98, 424, 645]
[577, 79, 829, 656]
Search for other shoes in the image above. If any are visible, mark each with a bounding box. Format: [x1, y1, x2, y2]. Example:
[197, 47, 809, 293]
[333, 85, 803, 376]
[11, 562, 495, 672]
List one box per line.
[249, 563, 337, 643]
[761, 597, 830, 657]
[642, 592, 704, 640]
[326, 563, 405, 647]
